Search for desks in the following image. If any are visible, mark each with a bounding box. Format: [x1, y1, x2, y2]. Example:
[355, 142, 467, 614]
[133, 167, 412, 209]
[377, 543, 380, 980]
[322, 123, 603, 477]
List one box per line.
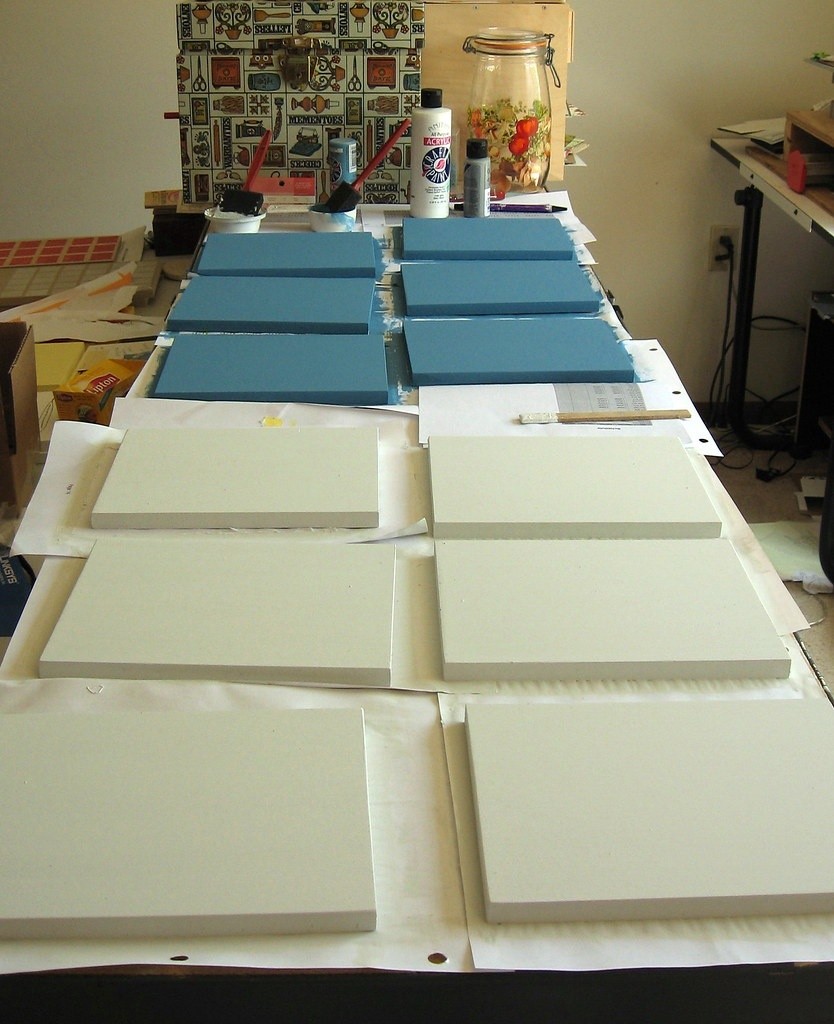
[710, 136, 834, 431]
[0, 204, 834, 1024]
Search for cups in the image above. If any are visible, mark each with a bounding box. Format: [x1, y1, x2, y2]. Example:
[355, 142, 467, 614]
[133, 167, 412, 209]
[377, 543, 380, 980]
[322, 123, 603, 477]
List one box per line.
[466, 28, 552, 194]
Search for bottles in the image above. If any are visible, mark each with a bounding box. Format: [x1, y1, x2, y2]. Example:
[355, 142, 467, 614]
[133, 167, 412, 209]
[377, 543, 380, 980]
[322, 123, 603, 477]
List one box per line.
[410, 88, 451, 218]
[329, 137, 357, 200]
[463, 138, 491, 218]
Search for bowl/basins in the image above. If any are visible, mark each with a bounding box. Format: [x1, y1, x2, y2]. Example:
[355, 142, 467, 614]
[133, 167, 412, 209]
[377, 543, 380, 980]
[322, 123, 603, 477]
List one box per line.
[309, 203, 356, 232]
[204, 207, 266, 233]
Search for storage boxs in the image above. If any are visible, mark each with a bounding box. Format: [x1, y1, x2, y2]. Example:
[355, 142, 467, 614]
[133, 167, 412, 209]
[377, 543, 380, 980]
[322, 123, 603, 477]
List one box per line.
[0, 322, 42, 518]
[53, 358, 146, 426]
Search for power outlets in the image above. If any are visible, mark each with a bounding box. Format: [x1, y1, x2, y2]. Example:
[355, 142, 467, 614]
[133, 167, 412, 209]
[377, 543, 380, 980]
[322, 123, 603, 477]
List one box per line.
[709, 225, 740, 272]
